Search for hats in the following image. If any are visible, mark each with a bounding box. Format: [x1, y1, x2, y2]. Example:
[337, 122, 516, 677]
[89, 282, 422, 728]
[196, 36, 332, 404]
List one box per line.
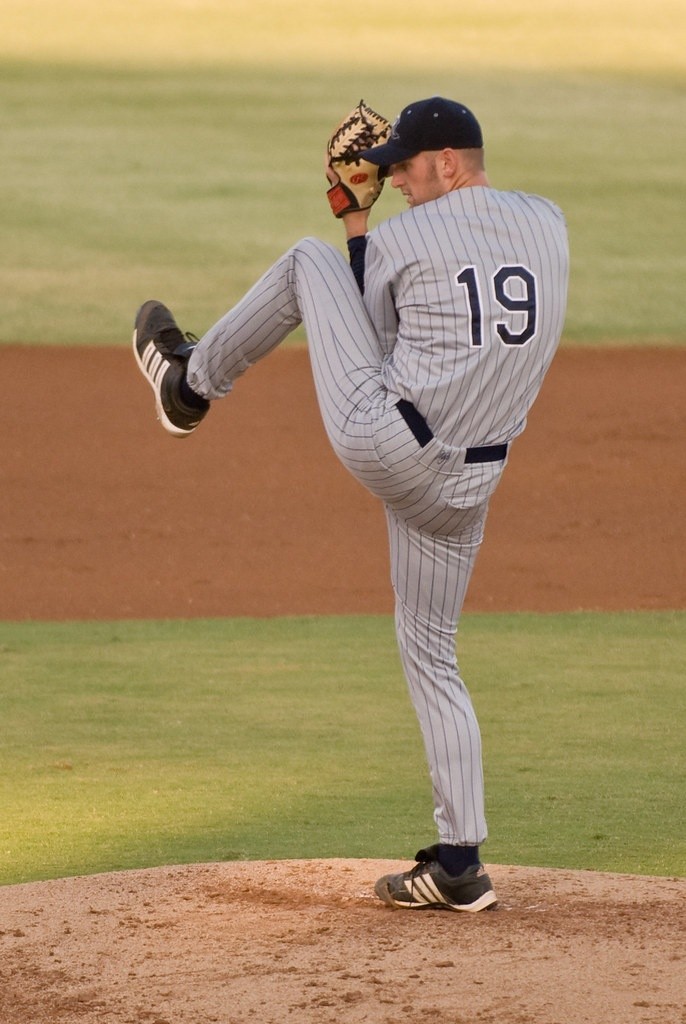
[358, 98, 483, 166]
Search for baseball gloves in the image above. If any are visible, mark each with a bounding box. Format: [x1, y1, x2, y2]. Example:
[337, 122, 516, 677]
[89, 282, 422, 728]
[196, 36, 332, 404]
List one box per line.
[324, 98, 395, 218]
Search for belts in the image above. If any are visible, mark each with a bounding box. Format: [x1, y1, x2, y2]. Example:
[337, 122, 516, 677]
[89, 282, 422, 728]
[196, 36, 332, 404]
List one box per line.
[395, 399, 507, 463]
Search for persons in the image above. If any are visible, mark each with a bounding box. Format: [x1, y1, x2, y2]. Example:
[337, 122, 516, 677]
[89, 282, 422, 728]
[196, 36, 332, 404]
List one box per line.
[133, 97, 570, 911]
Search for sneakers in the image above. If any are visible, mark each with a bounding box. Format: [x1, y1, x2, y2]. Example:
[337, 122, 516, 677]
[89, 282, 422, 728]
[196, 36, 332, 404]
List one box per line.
[375, 847, 498, 912]
[132, 300, 211, 437]
